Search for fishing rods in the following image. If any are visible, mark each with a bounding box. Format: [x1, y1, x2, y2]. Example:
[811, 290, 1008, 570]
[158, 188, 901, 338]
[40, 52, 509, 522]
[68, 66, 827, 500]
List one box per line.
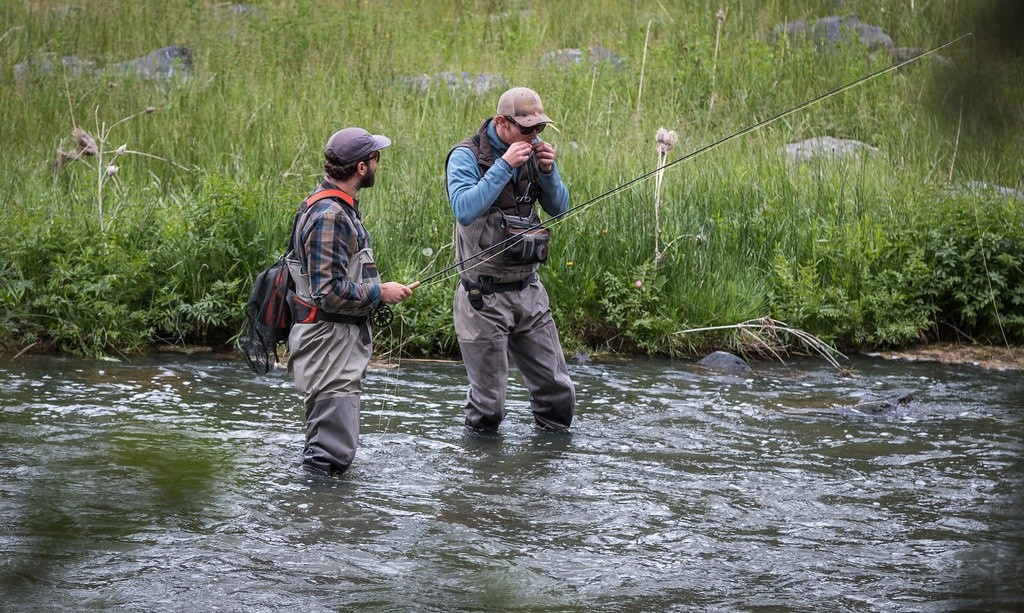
[370, 26, 974, 330]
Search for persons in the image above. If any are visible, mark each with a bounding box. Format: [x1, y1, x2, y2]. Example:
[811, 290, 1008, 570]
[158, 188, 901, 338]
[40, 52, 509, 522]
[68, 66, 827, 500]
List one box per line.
[443, 85, 576, 437]
[286, 127, 413, 477]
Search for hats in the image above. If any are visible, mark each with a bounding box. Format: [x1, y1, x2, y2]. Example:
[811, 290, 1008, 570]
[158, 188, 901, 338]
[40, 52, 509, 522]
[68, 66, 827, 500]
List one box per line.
[496, 87, 554, 127]
[323, 127, 392, 163]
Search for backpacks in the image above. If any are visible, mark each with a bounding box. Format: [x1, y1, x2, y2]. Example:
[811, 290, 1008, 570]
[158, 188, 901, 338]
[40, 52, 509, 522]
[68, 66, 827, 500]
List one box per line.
[245, 189, 361, 343]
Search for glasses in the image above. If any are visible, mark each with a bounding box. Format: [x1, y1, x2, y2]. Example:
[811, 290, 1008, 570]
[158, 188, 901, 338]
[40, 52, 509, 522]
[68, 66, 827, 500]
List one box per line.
[504, 115, 546, 135]
[356, 151, 380, 171]
